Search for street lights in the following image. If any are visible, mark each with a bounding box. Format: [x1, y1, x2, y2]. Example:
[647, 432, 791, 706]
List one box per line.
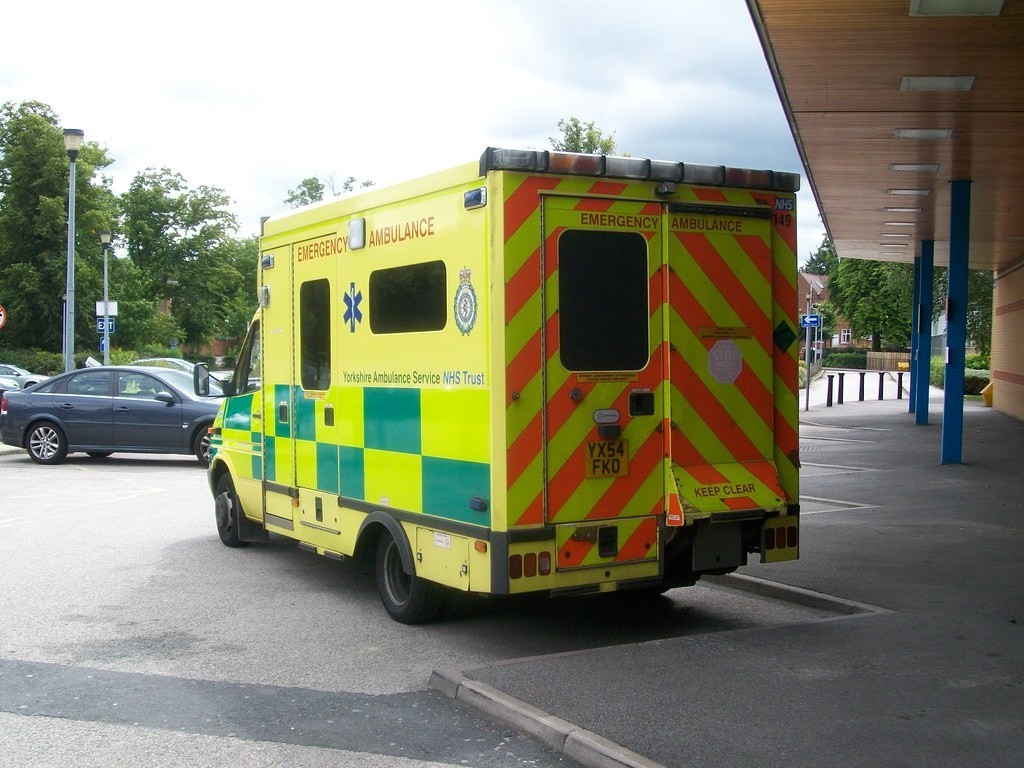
[60, 127, 85, 373]
[99, 227, 112, 368]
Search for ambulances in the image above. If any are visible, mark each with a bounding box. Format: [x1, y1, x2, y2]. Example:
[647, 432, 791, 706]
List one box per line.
[194, 146, 803, 625]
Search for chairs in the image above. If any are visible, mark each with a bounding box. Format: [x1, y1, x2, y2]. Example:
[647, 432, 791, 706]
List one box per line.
[120, 381, 127, 395]
[137, 379, 155, 397]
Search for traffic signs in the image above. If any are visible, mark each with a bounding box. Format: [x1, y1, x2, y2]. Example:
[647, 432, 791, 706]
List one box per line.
[800, 314, 818, 328]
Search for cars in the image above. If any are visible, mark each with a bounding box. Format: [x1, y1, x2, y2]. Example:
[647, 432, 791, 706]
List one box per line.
[123, 358, 226, 390]
[0, 364, 56, 391]
[0, 378, 19, 394]
[1, 365, 231, 466]
